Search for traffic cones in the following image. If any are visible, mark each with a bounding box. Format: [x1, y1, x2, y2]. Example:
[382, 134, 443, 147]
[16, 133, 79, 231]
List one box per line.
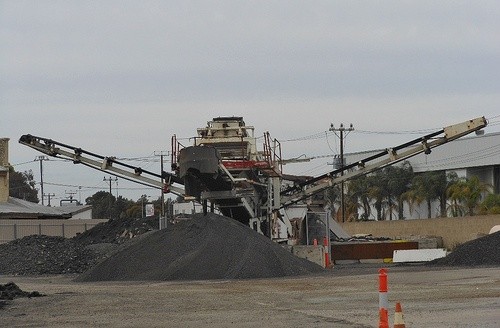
[394, 303, 404, 328]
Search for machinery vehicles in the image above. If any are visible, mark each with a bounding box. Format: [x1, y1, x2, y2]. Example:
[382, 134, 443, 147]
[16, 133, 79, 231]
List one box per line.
[0, 108, 486, 267]
